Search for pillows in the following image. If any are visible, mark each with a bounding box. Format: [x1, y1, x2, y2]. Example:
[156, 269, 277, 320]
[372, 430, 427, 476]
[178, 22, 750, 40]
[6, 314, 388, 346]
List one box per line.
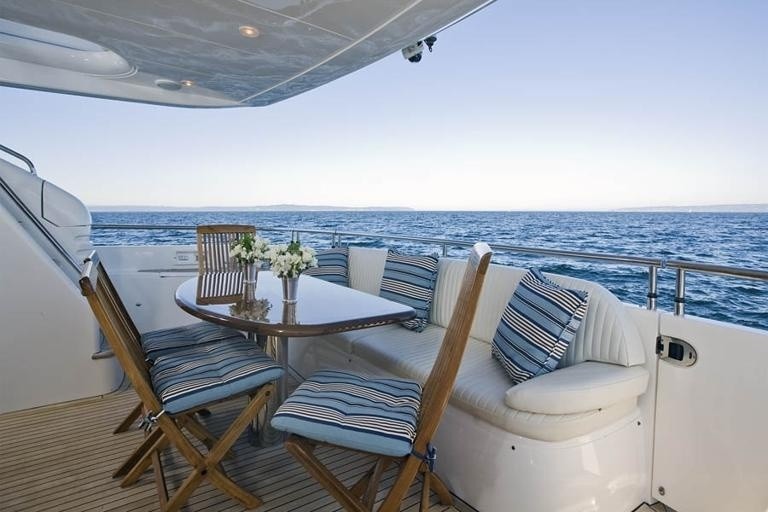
[491, 266, 588, 384]
[300, 248, 349, 287]
[377, 247, 442, 334]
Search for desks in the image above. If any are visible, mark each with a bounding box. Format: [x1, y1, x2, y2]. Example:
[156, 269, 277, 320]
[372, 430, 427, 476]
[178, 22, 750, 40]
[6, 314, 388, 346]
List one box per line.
[176, 271, 418, 442]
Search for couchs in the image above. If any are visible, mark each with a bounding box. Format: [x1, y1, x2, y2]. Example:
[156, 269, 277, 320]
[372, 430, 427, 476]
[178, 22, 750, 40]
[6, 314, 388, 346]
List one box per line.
[268, 245, 650, 512]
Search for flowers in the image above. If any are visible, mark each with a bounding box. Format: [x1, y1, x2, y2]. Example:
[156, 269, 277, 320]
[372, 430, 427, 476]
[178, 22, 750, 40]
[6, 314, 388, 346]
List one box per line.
[229, 298, 271, 318]
[269, 244, 319, 274]
[228, 235, 270, 264]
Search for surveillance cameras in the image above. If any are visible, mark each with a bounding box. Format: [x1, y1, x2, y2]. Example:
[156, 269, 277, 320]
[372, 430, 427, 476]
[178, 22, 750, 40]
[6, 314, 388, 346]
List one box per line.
[402, 42, 424, 63]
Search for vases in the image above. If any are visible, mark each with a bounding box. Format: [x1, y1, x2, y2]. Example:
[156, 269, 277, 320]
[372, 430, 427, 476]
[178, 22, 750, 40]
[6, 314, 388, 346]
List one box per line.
[242, 282, 256, 300]
[281, 277, 300, 302]
[282, 304, 298, 324]
[243, 264, 257, 282]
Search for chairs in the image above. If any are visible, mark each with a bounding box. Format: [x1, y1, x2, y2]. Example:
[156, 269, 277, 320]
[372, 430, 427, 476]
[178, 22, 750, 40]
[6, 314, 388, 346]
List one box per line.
[270, 242, 491, 509]
[83, 248, 249, 479]
[80, 262, 290, 508]
[196, 224, 258, 271]
[195, 271, 258, 305]
